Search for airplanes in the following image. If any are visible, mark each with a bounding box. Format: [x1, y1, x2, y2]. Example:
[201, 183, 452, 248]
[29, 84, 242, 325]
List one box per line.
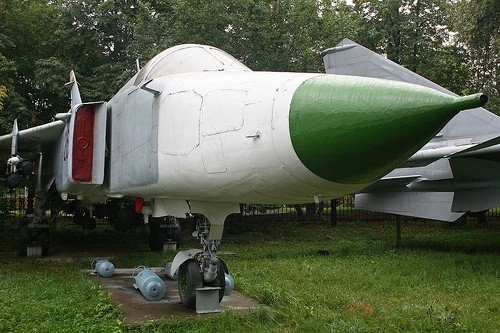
[0, 37, 500, 308]
